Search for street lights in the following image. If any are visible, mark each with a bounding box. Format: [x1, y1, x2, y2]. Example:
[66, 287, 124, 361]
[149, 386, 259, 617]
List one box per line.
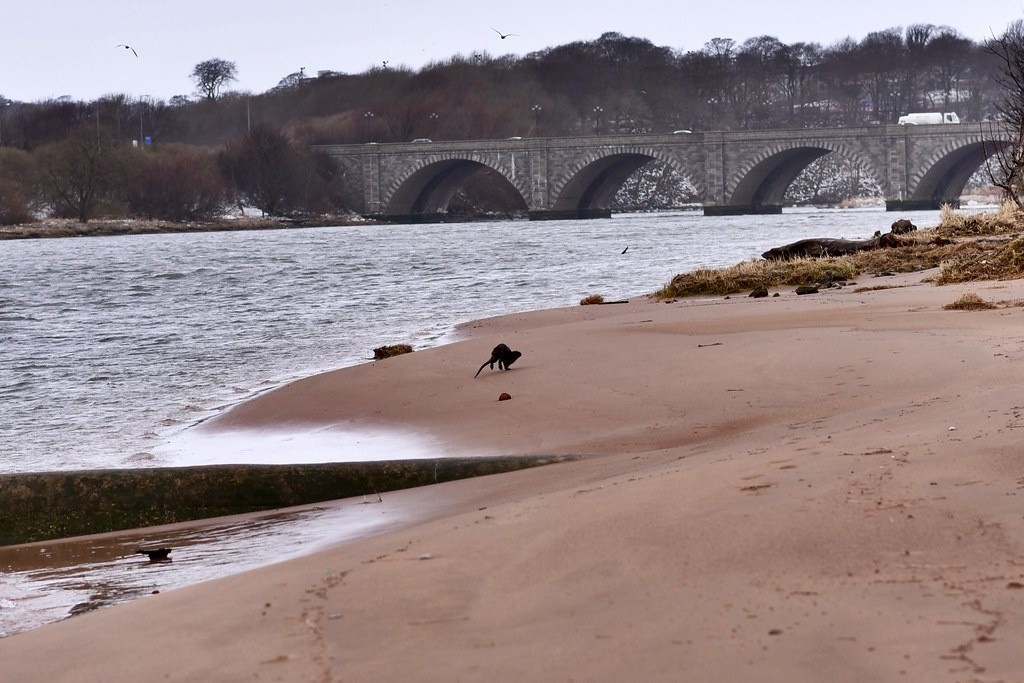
[890, 91, 900, 121]
[430, 113, 438, 140]
[364, 112, 374, 144]
[593, 106, 603, 135]
[532, 104, 542, 137]
[707, 97, 718, 132]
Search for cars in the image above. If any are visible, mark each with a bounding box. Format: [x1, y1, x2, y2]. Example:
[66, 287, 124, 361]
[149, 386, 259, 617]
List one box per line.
[674, 130, 692, 135]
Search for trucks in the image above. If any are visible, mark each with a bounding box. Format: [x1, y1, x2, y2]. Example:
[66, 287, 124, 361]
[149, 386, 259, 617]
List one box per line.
[898, 112, 960, 126]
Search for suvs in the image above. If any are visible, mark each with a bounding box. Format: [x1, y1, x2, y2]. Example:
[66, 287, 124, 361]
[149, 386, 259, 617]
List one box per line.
[411, 139, 433, 143]
